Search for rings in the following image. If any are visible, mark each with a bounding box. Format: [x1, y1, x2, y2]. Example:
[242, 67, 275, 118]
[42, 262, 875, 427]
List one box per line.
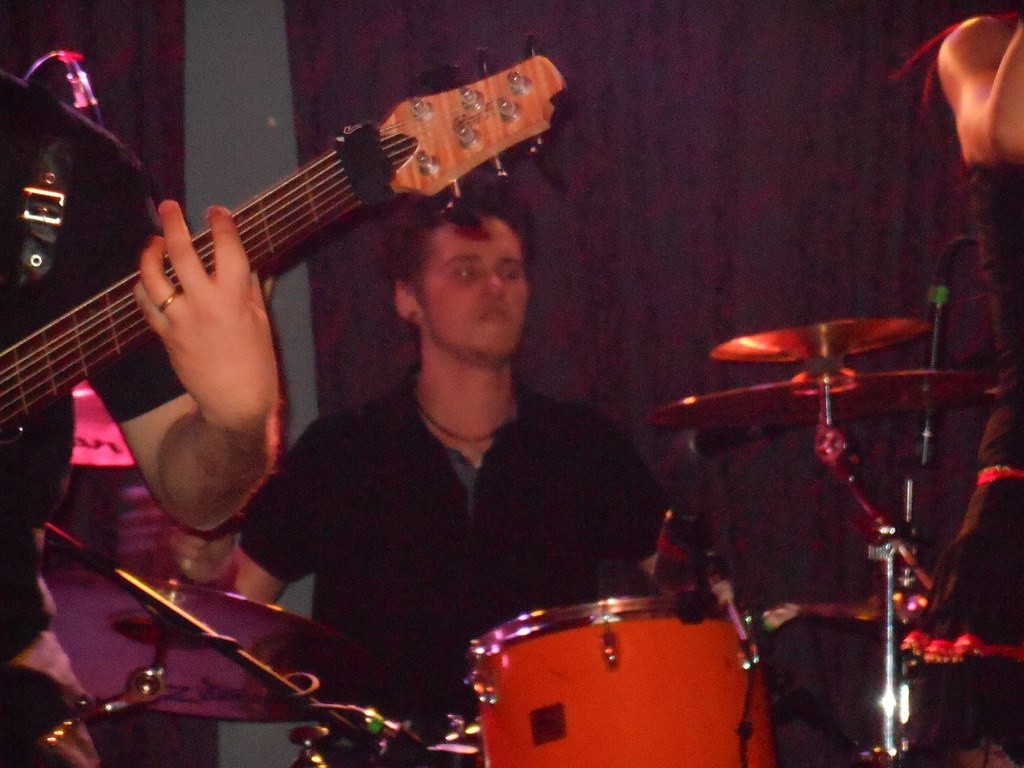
[157, 288, 182, 314]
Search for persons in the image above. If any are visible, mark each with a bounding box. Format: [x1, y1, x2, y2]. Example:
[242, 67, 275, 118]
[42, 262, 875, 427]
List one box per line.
[884, 1, 1022, 768]
[163, 174, 670, 767]
[0, 63, 287, 768]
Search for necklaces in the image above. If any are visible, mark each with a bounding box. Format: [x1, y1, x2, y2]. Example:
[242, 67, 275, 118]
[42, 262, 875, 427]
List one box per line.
[413, 397, 514, 443]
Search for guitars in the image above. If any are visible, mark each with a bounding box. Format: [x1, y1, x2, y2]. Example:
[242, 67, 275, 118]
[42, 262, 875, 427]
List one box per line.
[1, 38, 570, 451]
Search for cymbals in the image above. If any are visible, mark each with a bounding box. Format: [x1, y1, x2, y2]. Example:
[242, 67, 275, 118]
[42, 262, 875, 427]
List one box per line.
[705, 314, 932, 364]
[12, 557, 381, 722]
[641, 366, 990, 429]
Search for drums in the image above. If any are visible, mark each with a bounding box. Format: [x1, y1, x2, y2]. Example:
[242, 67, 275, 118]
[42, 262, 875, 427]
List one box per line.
[759, 595, 916, 739]
[465, 595, 780, 768]
